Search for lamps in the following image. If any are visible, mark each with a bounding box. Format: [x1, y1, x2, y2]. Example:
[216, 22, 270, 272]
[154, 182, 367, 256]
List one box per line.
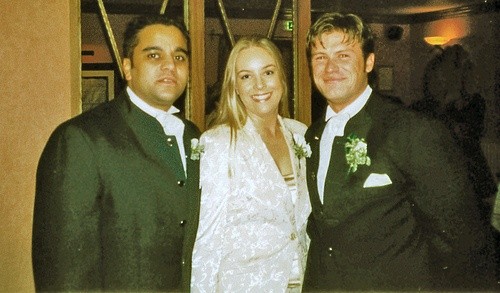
[424, 36, 451, 52]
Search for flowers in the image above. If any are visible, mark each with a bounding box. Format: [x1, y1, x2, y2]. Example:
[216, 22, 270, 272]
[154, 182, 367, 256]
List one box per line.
[344, 135, 372, 176]
[191, 138, 206, 160]
[293, 134, 312, 169]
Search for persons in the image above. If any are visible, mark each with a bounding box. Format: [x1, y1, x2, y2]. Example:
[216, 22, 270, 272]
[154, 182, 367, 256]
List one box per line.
[302, 13, 484, 293]
[191, 35, 312, 293]
[31, 13, 202, 293]
